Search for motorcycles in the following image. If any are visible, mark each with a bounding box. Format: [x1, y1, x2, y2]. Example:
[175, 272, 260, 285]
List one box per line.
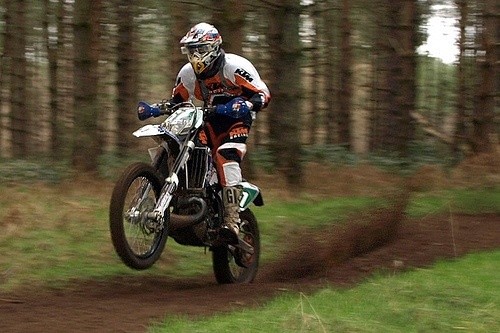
[109, 92, 264, 284]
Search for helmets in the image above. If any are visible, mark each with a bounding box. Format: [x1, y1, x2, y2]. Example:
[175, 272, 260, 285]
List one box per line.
[180, 22, 223, 74]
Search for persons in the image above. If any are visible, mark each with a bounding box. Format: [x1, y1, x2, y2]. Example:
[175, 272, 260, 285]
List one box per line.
[147, 23, 271, 246]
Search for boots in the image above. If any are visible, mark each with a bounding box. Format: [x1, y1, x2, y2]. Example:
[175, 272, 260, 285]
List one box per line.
[219, 185, 239, 246]
